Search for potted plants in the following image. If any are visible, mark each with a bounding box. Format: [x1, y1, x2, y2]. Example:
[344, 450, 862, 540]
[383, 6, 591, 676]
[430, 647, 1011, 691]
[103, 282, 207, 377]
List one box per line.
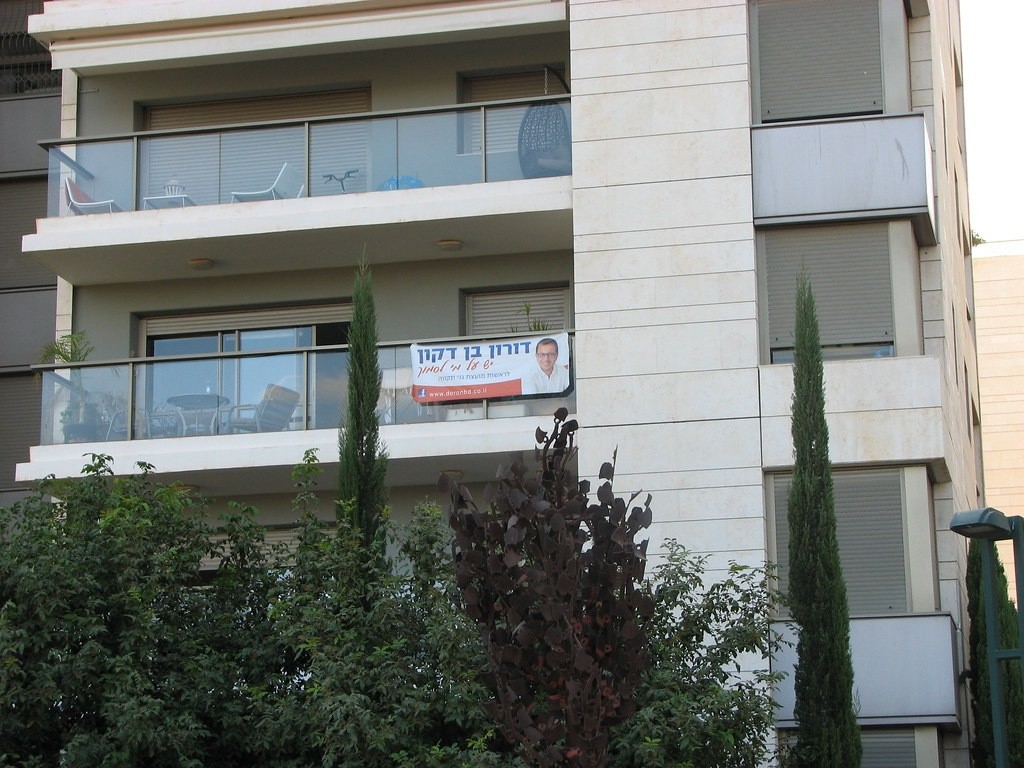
[33, 331, 101, 444]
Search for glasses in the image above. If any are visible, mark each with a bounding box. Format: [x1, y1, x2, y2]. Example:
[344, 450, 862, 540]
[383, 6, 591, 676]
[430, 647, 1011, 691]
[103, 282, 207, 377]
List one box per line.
[537, 352, 557, 358]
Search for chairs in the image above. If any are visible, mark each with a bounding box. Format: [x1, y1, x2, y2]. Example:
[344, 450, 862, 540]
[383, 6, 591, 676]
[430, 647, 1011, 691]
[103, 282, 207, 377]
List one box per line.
[230, 161, 304, 203]
[101, 393, 173, 441]
[224, 384, 303, 435]
[64, 177, 127, 216]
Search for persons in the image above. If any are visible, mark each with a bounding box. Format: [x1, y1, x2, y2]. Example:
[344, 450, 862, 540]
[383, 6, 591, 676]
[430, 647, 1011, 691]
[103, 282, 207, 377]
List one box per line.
[522, 338, 569, 393]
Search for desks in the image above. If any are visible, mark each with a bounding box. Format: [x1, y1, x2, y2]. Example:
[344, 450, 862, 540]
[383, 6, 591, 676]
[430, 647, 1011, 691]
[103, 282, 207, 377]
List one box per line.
[143, 195, 200, 212]
[167, 393, 229, 437]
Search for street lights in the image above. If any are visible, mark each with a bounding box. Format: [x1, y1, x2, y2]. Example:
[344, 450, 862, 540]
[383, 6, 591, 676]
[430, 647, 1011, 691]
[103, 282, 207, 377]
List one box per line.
[949, 508, 1024, 768]
